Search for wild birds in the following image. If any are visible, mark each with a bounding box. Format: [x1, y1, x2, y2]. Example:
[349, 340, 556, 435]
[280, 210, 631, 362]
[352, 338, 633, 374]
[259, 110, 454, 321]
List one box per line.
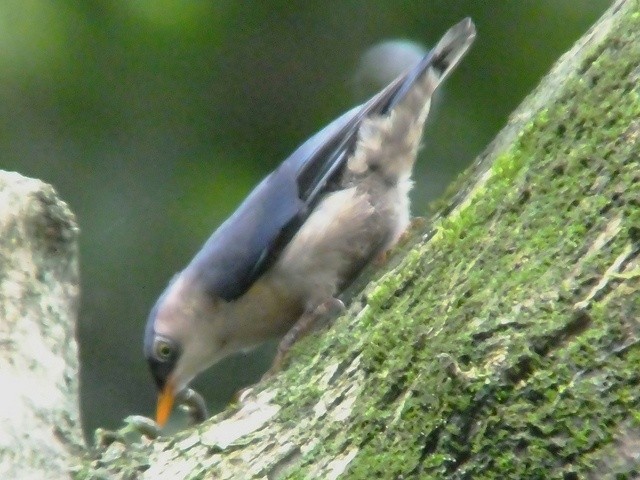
[143, 15, 476, 430]
